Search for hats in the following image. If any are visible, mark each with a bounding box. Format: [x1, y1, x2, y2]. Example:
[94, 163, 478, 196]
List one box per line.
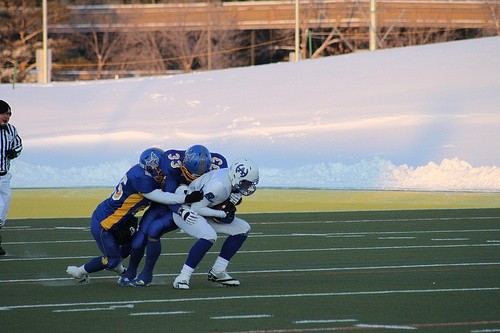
[0, 100, 11, 116]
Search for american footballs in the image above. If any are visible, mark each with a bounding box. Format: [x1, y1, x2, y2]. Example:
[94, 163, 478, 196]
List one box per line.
[211, 203, 228, 224]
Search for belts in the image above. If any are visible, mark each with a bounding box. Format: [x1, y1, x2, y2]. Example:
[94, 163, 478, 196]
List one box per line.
[0, 171, 7, 176]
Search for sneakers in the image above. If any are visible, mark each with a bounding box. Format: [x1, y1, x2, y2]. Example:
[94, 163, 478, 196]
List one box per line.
[173, 276, 190, 289]
[118, 269, 138, 286]
[208, 269, 240, 286]
[66, 266, 89, 286]
[112, 264, 127, 275]
[129, 273, 153, 287]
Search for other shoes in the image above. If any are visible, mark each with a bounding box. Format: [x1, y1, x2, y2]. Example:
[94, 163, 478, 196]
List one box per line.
[0, 246, 6, 255]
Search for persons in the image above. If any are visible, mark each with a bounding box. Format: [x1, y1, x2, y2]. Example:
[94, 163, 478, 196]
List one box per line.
[65, 145, 259, 290]
[0, 100, 23, 256]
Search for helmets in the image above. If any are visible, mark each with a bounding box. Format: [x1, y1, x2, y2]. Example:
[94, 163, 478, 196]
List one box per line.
[230, 157, 259, 196]
[139, 147, 165, 183]
[180, 145, 211, 183]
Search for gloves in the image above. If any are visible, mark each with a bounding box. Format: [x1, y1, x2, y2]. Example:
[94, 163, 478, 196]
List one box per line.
[177, 207, 198, 225]
[224, 200, 237, 225]
[185, 190, 203, 203]
[6, 150, 17, 159]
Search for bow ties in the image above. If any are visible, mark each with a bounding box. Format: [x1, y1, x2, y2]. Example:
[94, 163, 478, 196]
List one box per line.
[0, 125, 7, 129]
